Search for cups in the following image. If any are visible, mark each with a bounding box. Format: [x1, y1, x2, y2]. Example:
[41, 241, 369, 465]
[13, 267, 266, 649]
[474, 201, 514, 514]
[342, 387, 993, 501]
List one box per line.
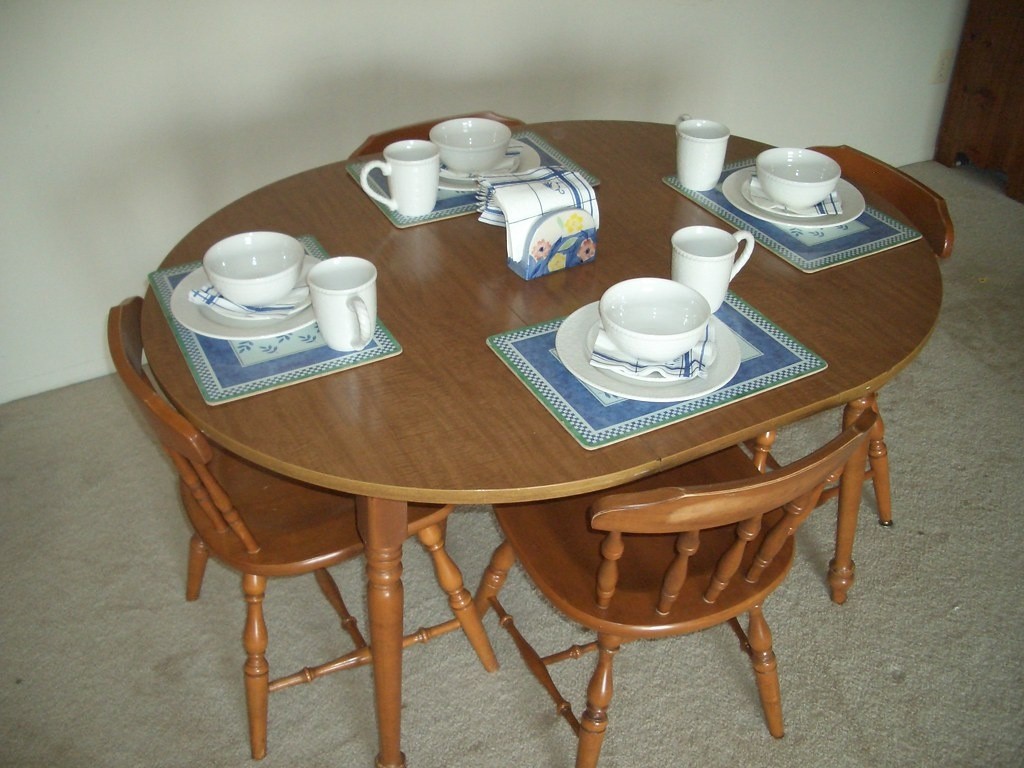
[307, 254, 378, 351]
[361, 140, 441, 218]
[428, 117, 511, 175]
[671, 225, 755, 315]
[674, 112, 731, 191]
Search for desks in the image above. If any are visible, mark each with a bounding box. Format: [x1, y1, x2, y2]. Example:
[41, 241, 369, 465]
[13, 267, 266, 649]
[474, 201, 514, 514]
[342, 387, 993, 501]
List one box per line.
[137, 120, 945, 768]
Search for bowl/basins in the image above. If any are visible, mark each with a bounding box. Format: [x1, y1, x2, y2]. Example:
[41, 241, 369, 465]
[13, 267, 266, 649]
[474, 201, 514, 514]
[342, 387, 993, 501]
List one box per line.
[203, 232, 304, 309]
[757, 147, 841, 208]
[599, 278, 711, 364]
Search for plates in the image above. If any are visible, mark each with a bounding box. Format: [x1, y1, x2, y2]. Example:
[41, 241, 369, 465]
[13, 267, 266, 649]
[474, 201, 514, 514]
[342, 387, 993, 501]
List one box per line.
[555, 301, 742, 402]
[434, 139, 540, 191]
[723, 167, 865, 228]
[171, 256, 319, 340]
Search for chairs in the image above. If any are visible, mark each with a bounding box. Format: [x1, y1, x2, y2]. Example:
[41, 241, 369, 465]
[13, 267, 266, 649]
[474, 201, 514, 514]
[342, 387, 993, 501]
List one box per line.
[106, 295, 498, 761]
[472, 406, 879, 768]
[742, 143, 956, 526]
